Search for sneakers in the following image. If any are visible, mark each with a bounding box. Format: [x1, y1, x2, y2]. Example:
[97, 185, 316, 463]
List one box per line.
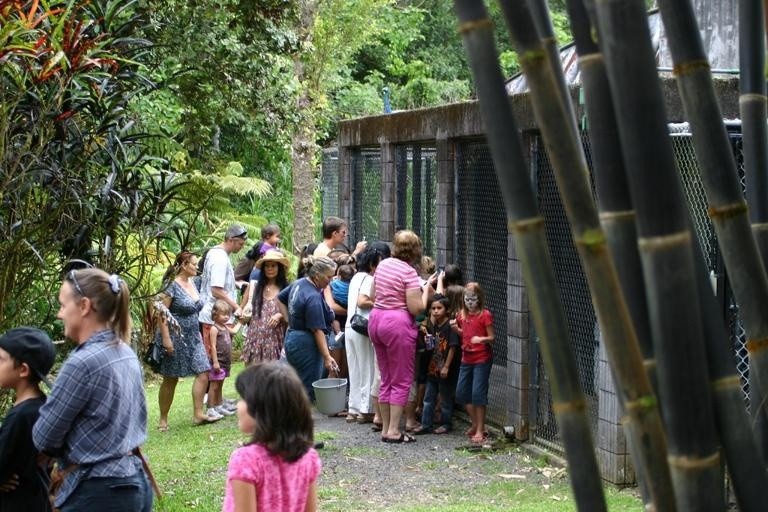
[222, 399, 236, 405]
[434, 425, 453, 434]
[205, 408, 225, 419]
[221, 403, 237, 411]
[414, 426, 432, 435]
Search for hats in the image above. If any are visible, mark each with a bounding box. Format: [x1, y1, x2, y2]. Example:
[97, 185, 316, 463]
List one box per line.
[256, 248, 290, 270]
[1, 327, 57, 390]
[226, 224, 248, 237]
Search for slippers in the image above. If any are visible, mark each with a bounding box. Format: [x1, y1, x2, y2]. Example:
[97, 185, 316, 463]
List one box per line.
[372, 427, 384, 432]
[386, 433, 416, 442]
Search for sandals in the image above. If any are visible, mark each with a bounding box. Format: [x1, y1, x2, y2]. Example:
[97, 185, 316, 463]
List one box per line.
[406, 423, 423, 433]
[193, 416, 224, 425]
[464, 430, 476, 435]
[345, 413, 359, 423]
[158, 424, 170, 431]
[356, 416, 374, 423]
[471, 434, 484, 443]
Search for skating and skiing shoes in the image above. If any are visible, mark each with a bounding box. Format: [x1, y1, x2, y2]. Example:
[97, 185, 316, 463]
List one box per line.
[214, 405, 235, 417]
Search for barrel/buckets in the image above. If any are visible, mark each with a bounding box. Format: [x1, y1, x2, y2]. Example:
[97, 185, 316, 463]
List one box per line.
[311, 377, 348, 414]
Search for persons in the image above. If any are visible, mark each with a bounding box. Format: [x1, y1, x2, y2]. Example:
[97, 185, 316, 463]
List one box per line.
[244, 252, 289, 362]
[450, 283, 496, 443]
[345, 249, 384, 423]
[296, 243, 317, 282]
[201, 225, 250, 404]
[413, 293, 459, 434]
[143, 252, 220, 432]
[233, 224, 281, 281]
[206, 302, 234, 420]
[328, 255, 357, 418]
[368, 231, 437, 443]
[31, 268, 152, 511]
[222, 362, 322, 512]
[370, 242, 390, 260]
[330, 265, 354, 341]
[307, 217, 346, 276]
[0, 326, 57, 512]
[274, 256, 340, 449]
[413, 253, 464, 293]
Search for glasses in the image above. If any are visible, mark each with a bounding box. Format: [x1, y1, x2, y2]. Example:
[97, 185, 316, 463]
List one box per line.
[337, 229, 346, 234]
[65, 257, 96, 314]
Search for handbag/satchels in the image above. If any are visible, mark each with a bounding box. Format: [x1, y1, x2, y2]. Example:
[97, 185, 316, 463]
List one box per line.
[350, 275, 369, 336]
